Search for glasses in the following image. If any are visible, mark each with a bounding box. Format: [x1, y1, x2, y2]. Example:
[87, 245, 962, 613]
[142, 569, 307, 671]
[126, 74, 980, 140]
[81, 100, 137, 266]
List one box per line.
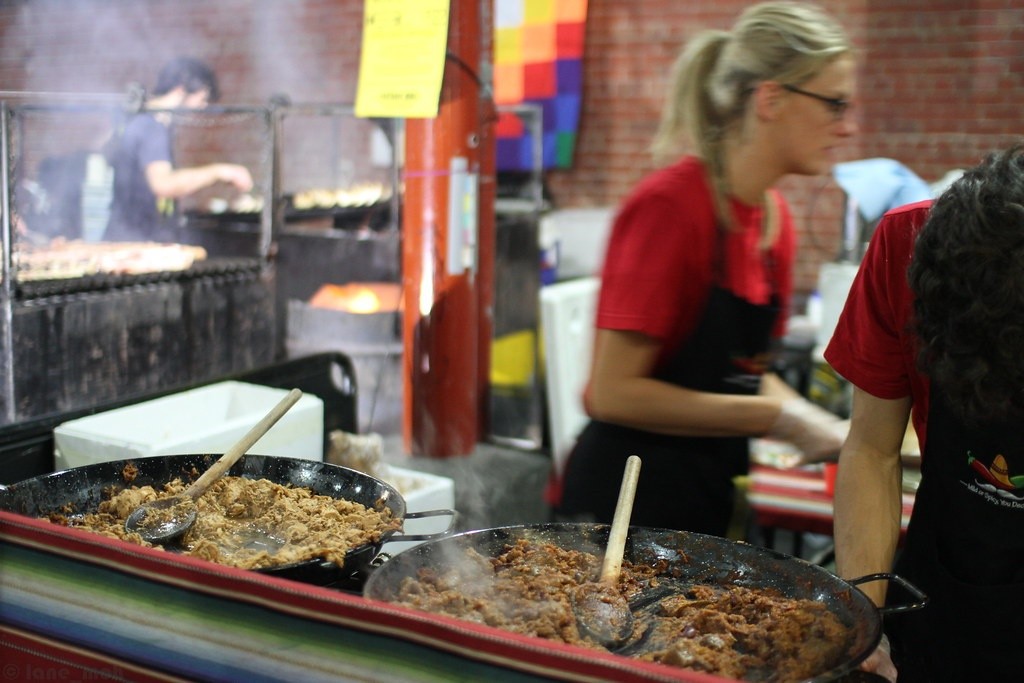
[782, 83, 851, 116]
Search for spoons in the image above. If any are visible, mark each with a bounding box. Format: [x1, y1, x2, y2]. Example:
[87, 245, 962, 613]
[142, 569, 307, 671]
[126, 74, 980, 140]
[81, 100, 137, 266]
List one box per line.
[575, 454, 642, 649]
[124, 386, 301, 547]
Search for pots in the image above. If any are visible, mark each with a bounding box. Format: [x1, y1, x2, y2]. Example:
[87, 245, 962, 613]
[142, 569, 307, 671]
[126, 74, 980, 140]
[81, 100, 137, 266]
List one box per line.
[362, 520, 930, 683]
[0, 452, 461, 589]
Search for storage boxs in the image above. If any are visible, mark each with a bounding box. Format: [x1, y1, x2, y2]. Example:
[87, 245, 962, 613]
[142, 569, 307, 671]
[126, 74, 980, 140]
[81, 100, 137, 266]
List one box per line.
[53, 381, 326, 471]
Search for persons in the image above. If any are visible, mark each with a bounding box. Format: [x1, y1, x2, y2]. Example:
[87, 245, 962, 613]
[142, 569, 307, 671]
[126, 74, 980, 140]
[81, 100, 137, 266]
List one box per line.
[824, 140, 1024, 683]
[81, 60, 254, 244]
[555, 1, 864, 540]
